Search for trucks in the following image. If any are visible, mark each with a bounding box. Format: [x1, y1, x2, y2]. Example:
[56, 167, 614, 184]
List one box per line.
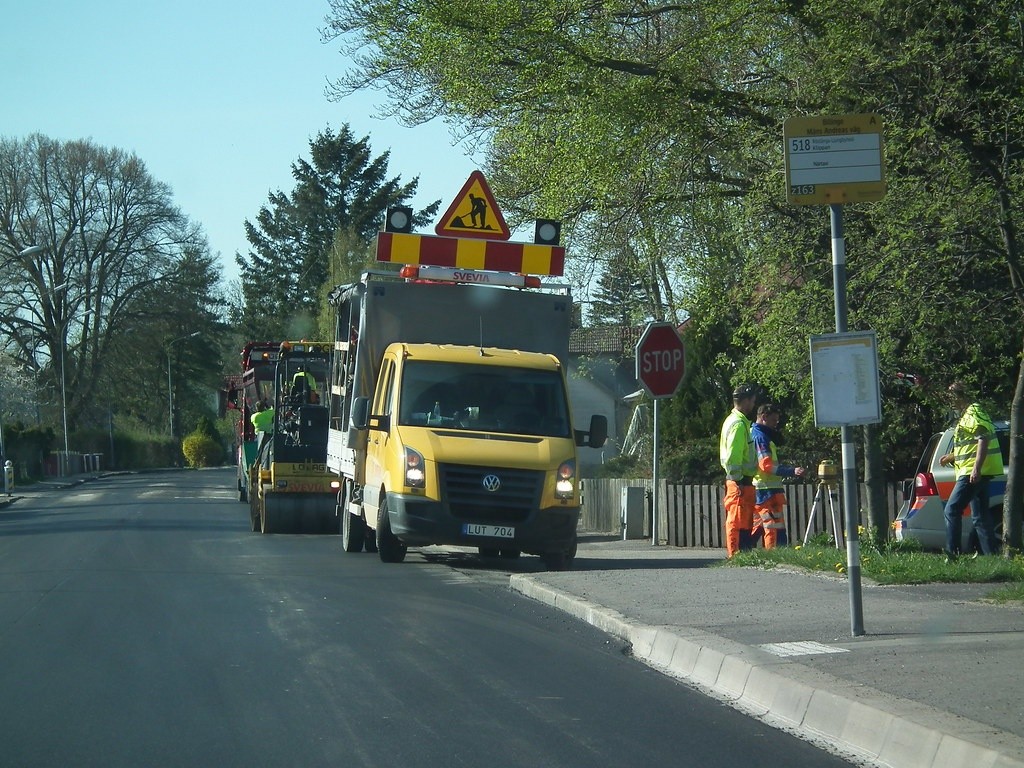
[227, 340, 331, 502]
[327, 266, 609, 570]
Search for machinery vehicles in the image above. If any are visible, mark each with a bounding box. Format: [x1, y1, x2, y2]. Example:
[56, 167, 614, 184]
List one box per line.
[242, 342, 351, 533]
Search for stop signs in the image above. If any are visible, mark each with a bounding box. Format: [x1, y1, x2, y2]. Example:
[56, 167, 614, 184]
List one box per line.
[635, 321, 686, 399]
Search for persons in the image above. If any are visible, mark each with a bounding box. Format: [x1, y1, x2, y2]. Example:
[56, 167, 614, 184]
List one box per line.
[249, 401, 275, 436]
[291, 365, 316, 401]
[719, 382, 758, 559]
[749, 403, 806, 548]
[939, 382, 1006, 563]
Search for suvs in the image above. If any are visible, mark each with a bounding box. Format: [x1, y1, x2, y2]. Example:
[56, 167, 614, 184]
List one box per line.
[895, 422, 1024, 553]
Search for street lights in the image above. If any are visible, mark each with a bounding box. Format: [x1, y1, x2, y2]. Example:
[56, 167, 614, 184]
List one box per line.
[32, 282, 72, 475]
[167, 331, 201, 439]
[62, 310, 96, 476]
